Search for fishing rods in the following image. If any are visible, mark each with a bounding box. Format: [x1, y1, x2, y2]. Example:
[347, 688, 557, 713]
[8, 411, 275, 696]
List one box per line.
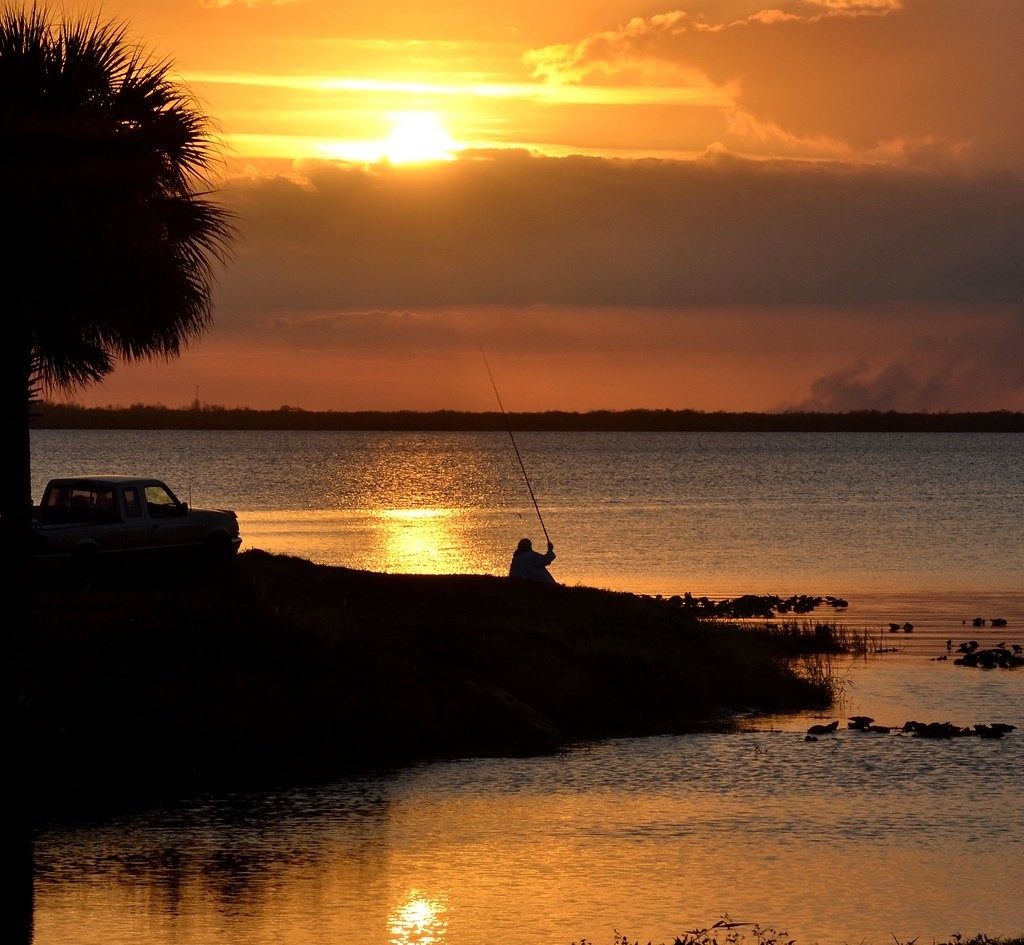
[474, 340, 553, 545]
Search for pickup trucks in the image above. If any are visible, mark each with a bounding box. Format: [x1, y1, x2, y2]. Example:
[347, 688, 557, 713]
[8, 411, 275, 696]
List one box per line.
[31, 477, 242, 569]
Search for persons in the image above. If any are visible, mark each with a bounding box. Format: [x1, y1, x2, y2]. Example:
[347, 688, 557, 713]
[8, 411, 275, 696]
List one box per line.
[509, 538, 561, 592]
